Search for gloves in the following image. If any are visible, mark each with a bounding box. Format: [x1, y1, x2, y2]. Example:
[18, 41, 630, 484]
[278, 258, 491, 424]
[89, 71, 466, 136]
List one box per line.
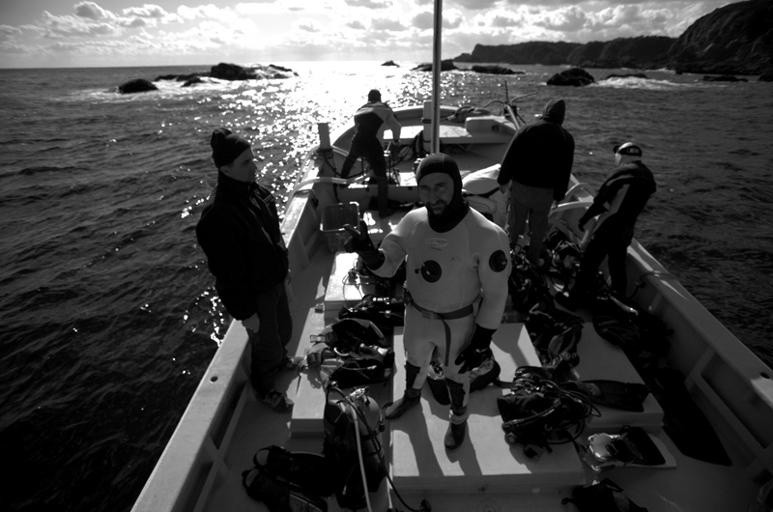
[342, 218, 384, 270]
[454, 323, 496, 374]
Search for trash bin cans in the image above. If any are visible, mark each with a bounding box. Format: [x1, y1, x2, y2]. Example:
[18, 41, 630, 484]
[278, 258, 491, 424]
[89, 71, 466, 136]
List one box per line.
[319, 201, 360, 253]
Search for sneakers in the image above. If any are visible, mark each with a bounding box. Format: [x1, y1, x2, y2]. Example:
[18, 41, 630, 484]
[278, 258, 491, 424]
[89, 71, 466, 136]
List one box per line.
[555, 291, 579, 312]
[282, 352, 295, 370]
[258, 389, 293, 414]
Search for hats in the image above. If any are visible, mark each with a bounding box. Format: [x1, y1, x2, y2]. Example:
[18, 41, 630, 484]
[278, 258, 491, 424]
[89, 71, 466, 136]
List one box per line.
[612, 141, 642, 156]
[210, 128, 250, 167]
[416, 152, 469, 233]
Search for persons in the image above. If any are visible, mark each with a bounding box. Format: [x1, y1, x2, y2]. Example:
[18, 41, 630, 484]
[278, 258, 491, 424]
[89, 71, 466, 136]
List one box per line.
[336, 89, 403, 218]
[555, 140, 658, 313]
[198, 127, 307, 413]
[497, 95, 575, 263]
[341, 153, 510, 448]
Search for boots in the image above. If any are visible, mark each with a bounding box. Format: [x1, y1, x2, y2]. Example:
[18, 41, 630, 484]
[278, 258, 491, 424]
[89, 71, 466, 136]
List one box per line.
[383, 387, 421, 419]
[444, 409, 468, 449]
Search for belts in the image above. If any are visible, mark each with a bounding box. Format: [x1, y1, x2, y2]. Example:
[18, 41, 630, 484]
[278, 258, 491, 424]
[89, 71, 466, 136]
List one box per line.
[404, 293, 474, 367]
